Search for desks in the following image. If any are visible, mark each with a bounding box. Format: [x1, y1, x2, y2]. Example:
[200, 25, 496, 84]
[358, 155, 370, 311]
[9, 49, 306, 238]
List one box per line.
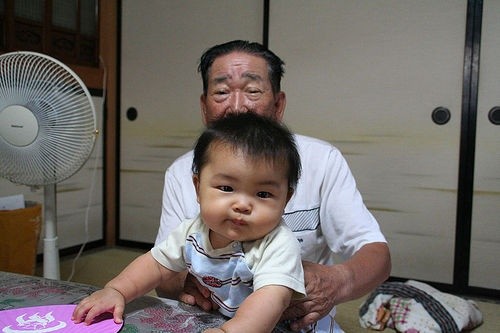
[0, 270, 229, 333]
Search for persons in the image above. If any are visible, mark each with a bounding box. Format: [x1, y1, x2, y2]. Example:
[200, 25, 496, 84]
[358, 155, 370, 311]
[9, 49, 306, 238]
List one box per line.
[70, 108, 307, 333]
[153, 39, 392, 333]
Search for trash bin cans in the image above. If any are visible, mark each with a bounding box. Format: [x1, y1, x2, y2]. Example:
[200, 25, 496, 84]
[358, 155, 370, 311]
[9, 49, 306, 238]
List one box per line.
[0, 195, 41, 276]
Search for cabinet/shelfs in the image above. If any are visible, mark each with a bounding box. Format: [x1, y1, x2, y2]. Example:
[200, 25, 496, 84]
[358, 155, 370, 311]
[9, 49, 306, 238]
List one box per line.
[97, 0, 500, 304]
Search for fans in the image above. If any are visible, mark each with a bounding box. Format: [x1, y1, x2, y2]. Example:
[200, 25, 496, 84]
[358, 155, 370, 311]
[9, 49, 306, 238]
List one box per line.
[0, 51, 100, 280]
